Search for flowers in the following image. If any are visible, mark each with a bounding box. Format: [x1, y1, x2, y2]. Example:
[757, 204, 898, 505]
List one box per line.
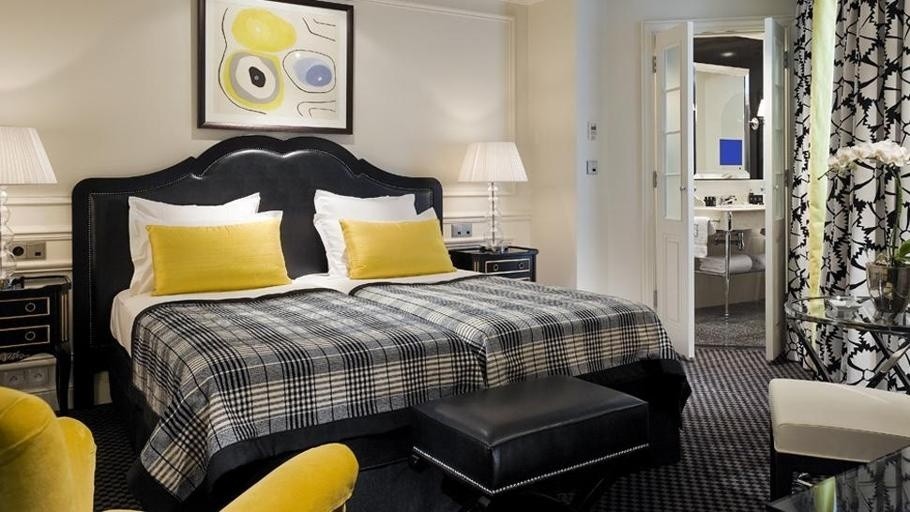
[816, 138, 910, 260]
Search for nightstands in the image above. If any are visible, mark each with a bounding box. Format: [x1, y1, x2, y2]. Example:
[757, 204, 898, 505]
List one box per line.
[450, 246, 539, 283]
[1, 276, 72, 417]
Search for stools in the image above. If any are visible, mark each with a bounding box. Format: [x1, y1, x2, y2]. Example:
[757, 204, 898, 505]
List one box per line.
[410, 373, 651, 512]
[767, 377, 910, 503]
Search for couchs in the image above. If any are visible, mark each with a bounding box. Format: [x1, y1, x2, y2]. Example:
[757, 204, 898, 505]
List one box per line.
[0, 388, 360, 512]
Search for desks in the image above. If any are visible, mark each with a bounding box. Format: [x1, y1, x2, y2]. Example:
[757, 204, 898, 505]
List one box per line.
[785, 295, 910, 393]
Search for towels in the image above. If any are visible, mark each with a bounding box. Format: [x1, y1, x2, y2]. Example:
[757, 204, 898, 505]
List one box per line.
[694, 216, 717, 258]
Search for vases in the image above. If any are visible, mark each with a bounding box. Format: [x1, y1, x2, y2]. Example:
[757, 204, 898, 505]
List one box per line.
[866, 259, 910, 315]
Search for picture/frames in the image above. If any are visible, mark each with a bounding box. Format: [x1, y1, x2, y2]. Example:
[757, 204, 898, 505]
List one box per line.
[198, 0, 353, 135]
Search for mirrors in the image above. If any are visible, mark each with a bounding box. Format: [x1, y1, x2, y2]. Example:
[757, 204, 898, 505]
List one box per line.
[694, 63, 752, 180]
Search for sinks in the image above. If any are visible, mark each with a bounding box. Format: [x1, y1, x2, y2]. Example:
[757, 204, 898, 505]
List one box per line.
[694, 205, 765, 231]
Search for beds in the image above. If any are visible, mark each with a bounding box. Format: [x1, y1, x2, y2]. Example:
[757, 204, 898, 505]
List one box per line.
[71, 136, 691, 512]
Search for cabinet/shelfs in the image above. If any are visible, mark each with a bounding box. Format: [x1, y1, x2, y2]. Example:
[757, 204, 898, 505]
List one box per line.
[694, 208, 765, 321]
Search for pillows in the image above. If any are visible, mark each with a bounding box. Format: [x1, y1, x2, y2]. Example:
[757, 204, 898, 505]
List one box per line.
[128, 192, 261, 216]
[128, 209, 284, 299]
[337, 219, 456, 280]
[312, 207, 438, 280]
[144, 218, 292, 296]
[314, 189, 415, 213]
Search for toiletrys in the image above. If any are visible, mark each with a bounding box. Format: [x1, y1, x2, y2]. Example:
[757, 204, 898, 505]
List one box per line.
[708, 197, 712, 206]
[712, 196, 716, 206]
[749, 189, 754, 203]
[704, 197, 707, 206]
[758, 195, 763, 204]
[754, 195, 757, 204]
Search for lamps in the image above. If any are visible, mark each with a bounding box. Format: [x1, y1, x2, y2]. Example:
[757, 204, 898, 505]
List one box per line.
[457, 141, 529, 251]
[1, 127, 58, 289]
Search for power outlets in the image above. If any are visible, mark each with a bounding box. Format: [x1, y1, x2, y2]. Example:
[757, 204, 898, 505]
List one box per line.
[452, 222, 472, 238]
[587, 160, 598, 176]
[26, 240, 46, 259]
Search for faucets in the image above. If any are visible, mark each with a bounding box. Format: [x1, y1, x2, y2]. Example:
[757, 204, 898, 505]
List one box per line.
[720, 194, 737, 204]
